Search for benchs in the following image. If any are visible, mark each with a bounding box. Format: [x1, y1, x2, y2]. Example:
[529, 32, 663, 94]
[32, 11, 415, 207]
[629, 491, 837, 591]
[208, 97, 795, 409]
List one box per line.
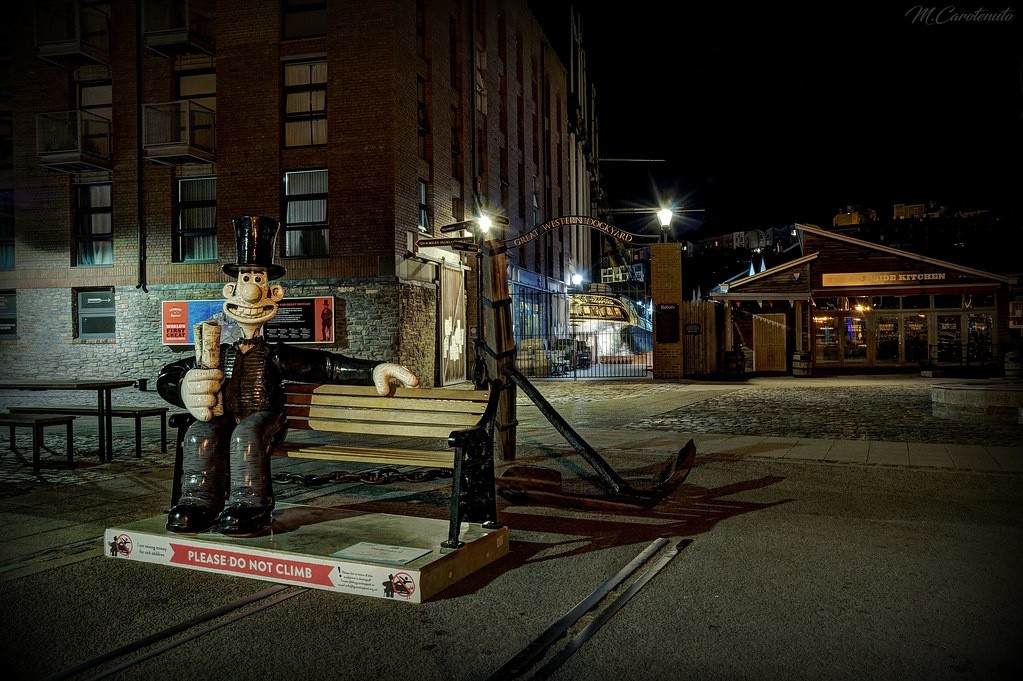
[163, 376, 505, 550]
[6, 403, 170, 459]
[0, 414, 78, 473]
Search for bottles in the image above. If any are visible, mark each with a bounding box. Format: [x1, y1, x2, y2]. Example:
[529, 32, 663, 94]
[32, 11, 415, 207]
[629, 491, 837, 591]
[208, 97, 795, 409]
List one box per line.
[880, 324, 894, 330]
[881, 331, 899, 340]
[817, 319, 834, 328]
[851, 325, 862, 331]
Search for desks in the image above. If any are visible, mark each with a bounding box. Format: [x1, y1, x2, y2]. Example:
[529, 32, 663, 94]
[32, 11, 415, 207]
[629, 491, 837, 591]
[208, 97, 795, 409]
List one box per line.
[0, 378, 137, 464]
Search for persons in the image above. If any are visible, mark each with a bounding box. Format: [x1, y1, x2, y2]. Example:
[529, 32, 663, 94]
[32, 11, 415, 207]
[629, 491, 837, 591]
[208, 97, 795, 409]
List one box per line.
[156, 216, 422, 539]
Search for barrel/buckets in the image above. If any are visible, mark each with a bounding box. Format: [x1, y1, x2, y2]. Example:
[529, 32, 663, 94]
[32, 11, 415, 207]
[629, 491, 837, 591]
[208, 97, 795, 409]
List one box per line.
[724, 350, 745, 381]
[793, 351, 812, 378]
[1004, 351, 1023, 377]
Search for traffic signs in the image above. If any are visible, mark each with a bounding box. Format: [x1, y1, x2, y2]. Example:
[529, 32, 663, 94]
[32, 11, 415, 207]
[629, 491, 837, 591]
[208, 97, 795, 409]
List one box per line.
[481, 209, 511, 226]
[440, 220, 474, 234]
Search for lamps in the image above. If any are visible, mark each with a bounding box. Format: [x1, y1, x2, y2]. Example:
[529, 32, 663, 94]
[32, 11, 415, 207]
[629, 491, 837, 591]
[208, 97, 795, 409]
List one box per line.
[478, 217, 493, 240]
[572, 274, 582, 284]
[655, 207, 675, 244]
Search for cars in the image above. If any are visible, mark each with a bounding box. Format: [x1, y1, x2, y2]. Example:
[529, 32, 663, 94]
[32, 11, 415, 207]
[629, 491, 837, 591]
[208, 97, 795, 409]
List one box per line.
[555, 337, 591, 369]
